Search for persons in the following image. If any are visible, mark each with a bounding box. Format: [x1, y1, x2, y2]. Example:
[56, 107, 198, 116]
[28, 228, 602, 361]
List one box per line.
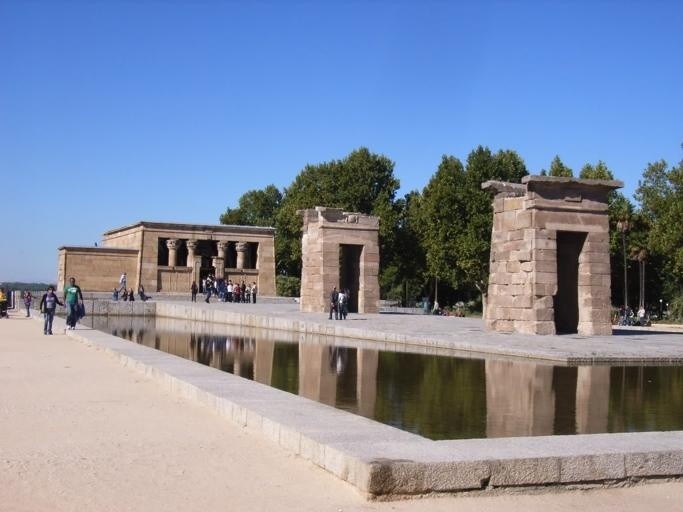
[190, 274, 256, 303]
[414, 296, 465, 316]
[0, 287, 11, 318]
[40, 287, 63, 335]
[24, 288, 36, 317]
[328, 346, 348, 375]
[63, 278, 83, 330]
[328, 287, 350, 320]
[189, 332, 255, 355]
[112, 273, 151, 301]
[113, 328, 144, 343]
[617, 305, 668, 326]
